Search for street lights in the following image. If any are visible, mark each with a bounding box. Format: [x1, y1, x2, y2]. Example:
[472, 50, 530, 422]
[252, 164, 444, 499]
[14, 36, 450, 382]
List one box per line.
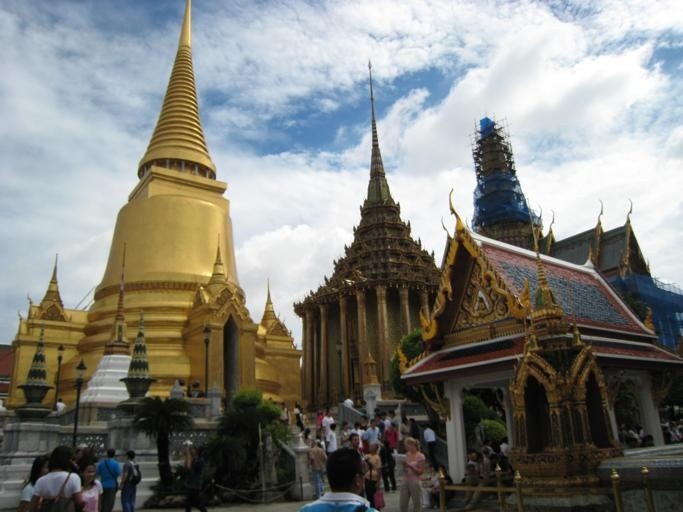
[53, 344, 64, 412]
[73, 358, 87, 448]
[203, 324, 211, 397]
[336, 338, 345, 402]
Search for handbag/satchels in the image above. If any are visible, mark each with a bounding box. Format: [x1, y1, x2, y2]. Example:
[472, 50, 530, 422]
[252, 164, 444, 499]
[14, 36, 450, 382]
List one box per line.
[47, 471, 77, 511]
[114, 481, 120, 493]
[373, 487, 387, 509]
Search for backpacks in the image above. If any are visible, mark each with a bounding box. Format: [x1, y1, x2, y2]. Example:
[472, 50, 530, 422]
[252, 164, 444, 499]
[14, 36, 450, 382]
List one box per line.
[126, 460, 142, 485]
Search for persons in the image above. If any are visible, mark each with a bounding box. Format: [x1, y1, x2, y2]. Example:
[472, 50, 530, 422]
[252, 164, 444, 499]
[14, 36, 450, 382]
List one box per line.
[17, 444, 142, 511]
[183, 445, 208, 512]
[276, 399, 514, 511]
[617, 420, 683, 448]
[56, 398, 66, 411]
